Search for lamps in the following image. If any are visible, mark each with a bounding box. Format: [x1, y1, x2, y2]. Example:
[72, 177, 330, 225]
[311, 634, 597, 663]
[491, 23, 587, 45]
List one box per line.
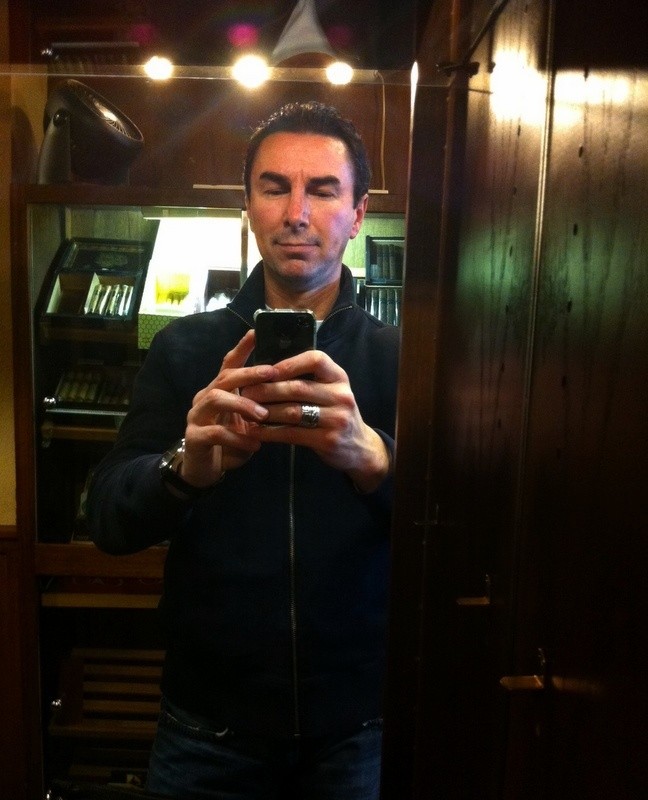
[38, 78, 145, 186]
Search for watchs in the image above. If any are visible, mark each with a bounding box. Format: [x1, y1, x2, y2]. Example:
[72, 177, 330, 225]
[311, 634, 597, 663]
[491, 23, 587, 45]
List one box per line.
[160, 437, 227, 498]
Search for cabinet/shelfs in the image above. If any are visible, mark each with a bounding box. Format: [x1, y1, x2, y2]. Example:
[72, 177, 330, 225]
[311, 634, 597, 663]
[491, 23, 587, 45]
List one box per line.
[9, 181, 407, 800]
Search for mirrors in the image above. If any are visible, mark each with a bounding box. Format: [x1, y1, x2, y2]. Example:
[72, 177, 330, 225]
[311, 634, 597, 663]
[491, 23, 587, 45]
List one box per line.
[0, 0, 557, 800]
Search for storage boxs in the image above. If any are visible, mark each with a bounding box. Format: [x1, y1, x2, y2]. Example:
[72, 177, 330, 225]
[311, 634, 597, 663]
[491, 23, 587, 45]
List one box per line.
[37, 209, 406, 431]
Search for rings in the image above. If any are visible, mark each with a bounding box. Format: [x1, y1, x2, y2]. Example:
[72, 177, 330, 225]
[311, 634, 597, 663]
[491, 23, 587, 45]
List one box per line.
[298, 403, 320, 430]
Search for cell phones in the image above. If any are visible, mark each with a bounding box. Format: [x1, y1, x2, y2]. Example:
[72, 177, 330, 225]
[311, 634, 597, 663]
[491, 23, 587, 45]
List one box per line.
[252, 308, 317, 384]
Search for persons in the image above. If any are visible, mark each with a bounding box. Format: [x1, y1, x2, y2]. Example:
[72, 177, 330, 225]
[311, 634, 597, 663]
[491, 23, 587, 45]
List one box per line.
[85, 102, 403, 800]
